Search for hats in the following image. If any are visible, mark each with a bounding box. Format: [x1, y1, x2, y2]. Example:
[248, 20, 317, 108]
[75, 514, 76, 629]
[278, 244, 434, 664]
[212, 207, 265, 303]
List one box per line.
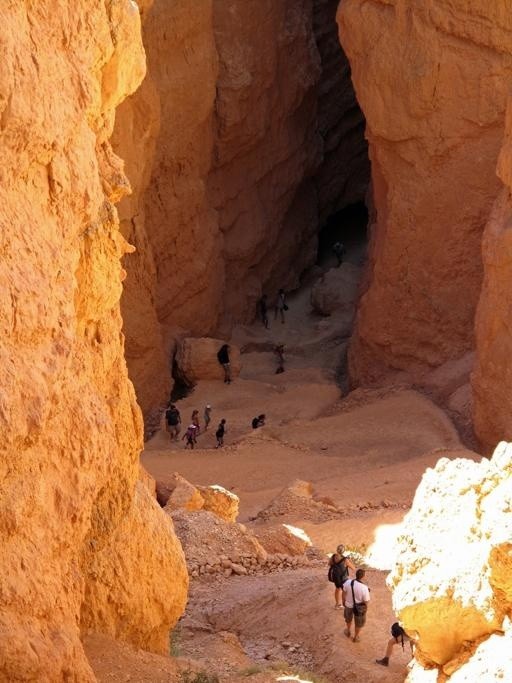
[170, 404, 176, 409]
[188, 423, 196, 428]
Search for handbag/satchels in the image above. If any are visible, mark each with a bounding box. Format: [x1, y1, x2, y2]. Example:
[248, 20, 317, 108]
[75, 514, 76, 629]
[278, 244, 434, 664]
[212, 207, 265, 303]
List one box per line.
[391, 622, 405, 638]
[352, 601, 367, 616]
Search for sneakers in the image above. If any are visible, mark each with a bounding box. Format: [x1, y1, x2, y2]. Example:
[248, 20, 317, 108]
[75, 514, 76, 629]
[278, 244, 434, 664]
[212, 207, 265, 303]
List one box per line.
[335, 605, 346, 612]
[344, 628, 351, 637]
[353, 636, 360, 642]
[375, 655, 390, 666]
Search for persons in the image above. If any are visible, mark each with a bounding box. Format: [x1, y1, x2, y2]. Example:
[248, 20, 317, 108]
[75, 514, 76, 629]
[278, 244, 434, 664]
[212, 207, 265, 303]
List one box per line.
[165, 404, 226, 449]
[332, 238, 346, 267]
[328, 544, 370, 642]
[257, 290, 288, 329]
[252, 414, 266, 429]
[218, 343, 232, 384]
[375, 622, 415, 666]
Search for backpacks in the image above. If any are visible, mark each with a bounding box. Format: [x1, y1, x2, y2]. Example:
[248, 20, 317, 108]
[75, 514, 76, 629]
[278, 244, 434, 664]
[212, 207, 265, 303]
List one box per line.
[327, 553, 350, 584]
